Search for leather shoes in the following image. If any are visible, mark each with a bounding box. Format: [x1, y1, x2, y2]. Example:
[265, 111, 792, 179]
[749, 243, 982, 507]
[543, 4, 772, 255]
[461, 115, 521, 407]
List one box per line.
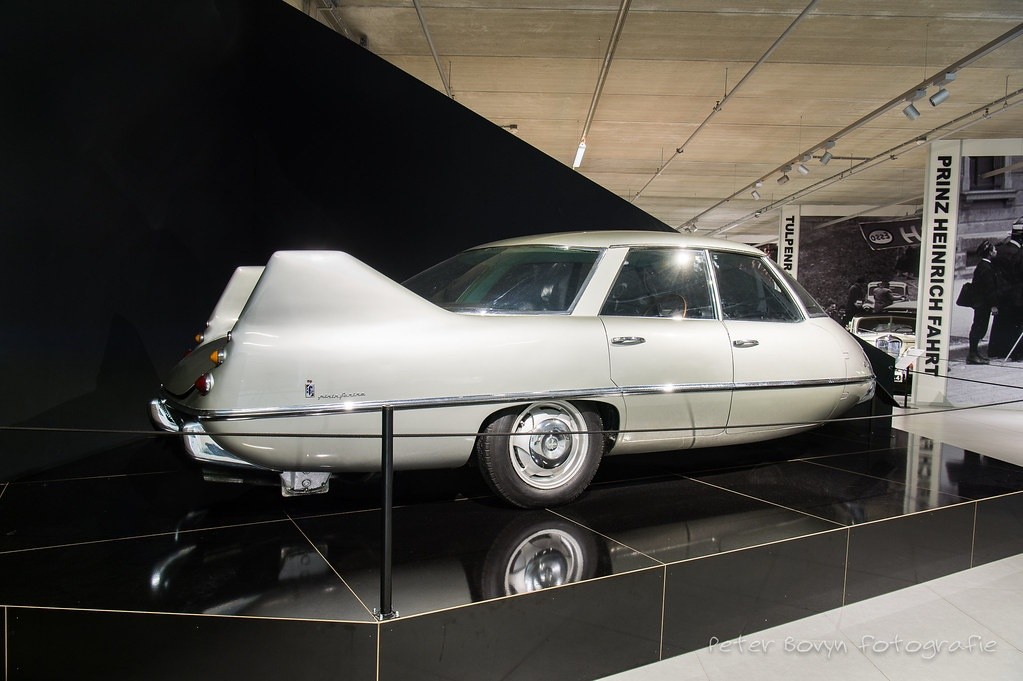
[977, 352, 990, 361]
[966, 355, 988, 365]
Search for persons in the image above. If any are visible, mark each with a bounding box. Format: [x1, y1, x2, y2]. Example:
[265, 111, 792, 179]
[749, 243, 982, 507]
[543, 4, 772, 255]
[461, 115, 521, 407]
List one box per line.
[825, 279, 894, 328]
[966, 215, 1023, 365]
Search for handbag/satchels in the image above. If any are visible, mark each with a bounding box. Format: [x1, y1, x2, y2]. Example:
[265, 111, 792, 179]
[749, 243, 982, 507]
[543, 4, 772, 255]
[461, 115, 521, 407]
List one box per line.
[956, 283, 975, 307]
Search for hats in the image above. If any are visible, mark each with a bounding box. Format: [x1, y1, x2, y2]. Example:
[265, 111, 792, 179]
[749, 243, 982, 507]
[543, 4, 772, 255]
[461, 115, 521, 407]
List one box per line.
[977, 239, 990, 255]
[1012, 217, 1023, 230]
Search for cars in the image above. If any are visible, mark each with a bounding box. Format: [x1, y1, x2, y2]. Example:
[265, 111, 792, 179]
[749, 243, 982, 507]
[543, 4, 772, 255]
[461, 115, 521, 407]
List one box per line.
[149, 230, 882, 514]
[849, 280, 917, 363]
[150, 437, 967, 621]
